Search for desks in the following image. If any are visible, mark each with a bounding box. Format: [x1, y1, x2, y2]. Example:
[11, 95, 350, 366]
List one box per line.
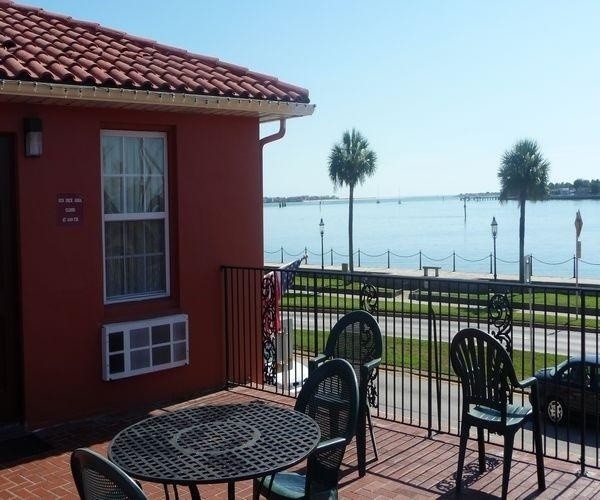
[108, 403, 321, 500]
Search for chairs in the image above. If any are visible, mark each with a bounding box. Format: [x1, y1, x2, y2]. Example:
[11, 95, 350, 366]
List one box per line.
[450, 328, 546, 500]
[306, 309, 383, 478]
[255, 358, 360, 500]
[70, 448, 150, 500]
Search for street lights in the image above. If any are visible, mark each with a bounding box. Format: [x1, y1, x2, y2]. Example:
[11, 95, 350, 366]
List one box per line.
[319, 218, 325, 287]
[490, 216, 498, 280]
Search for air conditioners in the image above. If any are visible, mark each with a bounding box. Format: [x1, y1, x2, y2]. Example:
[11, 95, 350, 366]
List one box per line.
[273, 318, 295, 373]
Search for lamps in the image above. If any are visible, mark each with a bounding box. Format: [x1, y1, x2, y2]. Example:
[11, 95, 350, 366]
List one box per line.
[23, 115, 45, 156]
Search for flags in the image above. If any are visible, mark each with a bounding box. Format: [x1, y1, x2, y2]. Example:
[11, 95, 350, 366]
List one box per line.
[262, 257, 303, 383]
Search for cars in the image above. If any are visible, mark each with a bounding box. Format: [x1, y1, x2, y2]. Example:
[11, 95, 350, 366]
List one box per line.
[529, 353, 600, 435]
